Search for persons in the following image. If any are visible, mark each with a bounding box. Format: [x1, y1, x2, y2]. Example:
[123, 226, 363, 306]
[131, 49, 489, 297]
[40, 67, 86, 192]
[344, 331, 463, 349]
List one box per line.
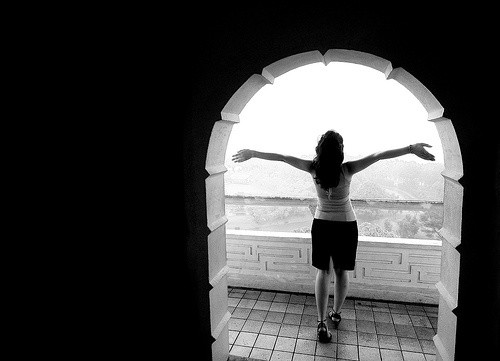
[232, 131, 436, 343]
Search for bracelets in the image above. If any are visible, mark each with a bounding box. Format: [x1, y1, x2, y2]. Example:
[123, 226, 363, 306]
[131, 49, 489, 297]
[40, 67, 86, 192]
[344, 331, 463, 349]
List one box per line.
[409, 145, 413, 153]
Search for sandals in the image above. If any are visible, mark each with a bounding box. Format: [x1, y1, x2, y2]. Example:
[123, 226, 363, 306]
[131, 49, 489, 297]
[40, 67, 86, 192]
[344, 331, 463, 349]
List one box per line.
[329, 309, 342, 328]
[317, 323, 332, 342]
